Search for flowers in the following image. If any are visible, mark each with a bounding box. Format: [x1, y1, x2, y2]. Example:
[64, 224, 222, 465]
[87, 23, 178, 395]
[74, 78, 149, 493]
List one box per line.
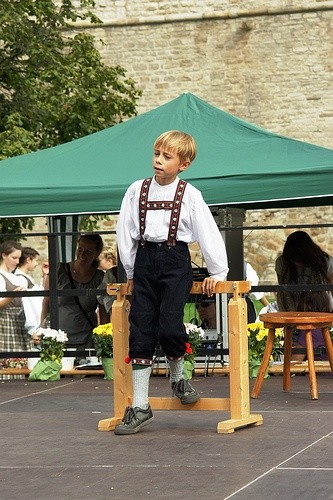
[247, 322, 282, 361]
[92, 323, 113, 358]
[35, 327, 69, 364]
[183, 322, 205, 361]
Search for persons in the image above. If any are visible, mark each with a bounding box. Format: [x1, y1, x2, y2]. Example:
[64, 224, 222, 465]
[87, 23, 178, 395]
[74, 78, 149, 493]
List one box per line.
[113, 130, 230, 435]
[193, 302, 217, 329]
[96, 242, 128, 323]
[1, 240, 31, 380]
[275, 231, 333, 361]
[35, 235, 110, 363]
[11, 247, 49, 289]
[242, 260, 270, 325]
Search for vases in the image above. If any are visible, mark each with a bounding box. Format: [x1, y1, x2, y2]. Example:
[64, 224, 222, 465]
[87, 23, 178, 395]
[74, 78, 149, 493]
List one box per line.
[101, 358, 114, 380]
[29, 360, 62, 380]
[184, 360, 193, 380]
[248, 361, 269, 379]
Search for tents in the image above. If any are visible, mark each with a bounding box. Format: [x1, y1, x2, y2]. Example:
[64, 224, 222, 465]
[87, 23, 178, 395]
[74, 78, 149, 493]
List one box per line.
[0, 91, 333, 282]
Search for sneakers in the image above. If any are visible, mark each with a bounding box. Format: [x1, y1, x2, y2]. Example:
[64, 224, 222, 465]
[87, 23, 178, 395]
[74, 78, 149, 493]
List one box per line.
[114, 404, 155, 435]
[172, 379, 200, 405]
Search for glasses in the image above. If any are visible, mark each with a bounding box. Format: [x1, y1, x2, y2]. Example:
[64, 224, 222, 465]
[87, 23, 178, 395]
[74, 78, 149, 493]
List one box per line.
[195, 302, 214, 309]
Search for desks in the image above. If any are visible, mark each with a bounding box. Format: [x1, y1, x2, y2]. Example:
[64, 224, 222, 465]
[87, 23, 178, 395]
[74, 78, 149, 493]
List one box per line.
[250, 311, 333, 400]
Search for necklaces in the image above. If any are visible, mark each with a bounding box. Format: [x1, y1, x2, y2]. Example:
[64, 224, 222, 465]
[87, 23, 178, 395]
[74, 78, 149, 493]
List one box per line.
[72, 260, 94, 283]
